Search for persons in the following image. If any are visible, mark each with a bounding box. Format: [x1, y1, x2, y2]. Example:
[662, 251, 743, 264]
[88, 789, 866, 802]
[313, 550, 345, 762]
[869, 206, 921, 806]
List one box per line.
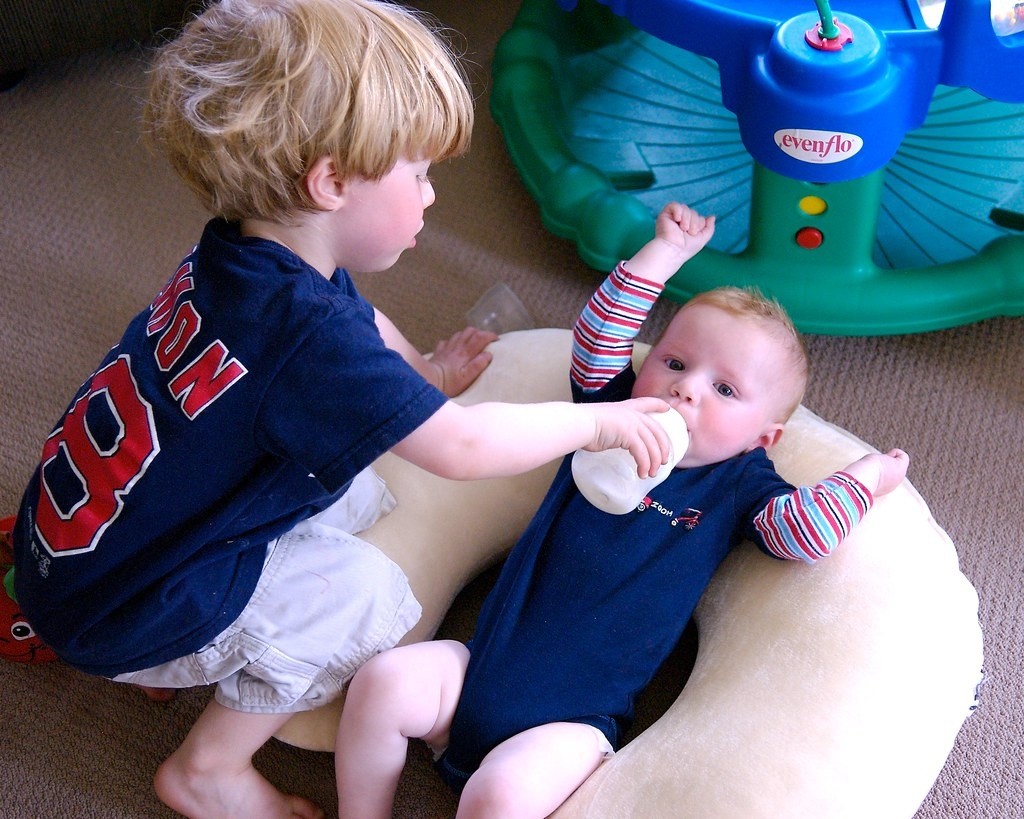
[334, 202, 911, 819]
[18, 2, 672, 818]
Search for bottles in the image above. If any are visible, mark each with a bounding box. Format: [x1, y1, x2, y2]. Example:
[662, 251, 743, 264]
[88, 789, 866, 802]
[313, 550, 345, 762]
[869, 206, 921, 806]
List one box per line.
[572, 405, 690, 515]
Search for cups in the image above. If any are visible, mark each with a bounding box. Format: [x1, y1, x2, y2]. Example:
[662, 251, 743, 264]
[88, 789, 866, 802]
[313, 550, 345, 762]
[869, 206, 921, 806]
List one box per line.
[466, 282, 535, 337]
